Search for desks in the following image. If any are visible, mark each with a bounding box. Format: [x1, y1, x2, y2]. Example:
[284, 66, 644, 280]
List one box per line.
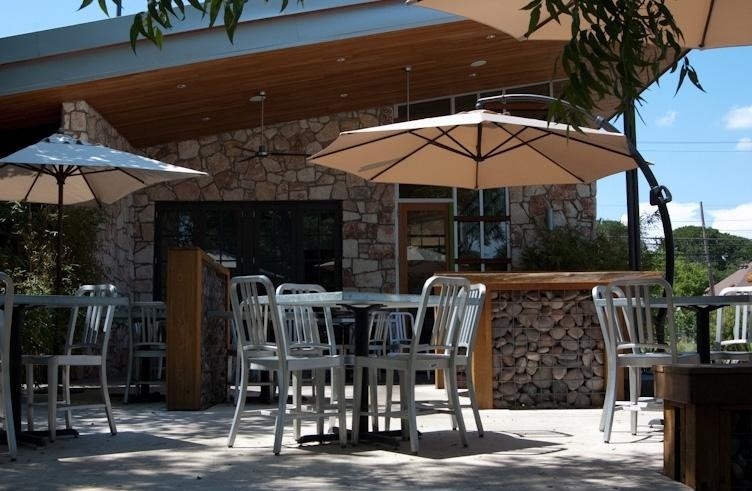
[597, 296, 750, 365]
[252, 292, 481, 449]
[0, 295, 127, 450]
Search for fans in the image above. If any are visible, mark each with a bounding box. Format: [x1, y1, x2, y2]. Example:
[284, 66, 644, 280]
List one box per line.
[231, 90, 311, 163]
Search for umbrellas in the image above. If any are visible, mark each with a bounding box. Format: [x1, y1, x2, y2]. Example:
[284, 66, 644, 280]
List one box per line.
[0, 130, 210, 295]
[306, 104, 655, 192]
[410, 0, 751, 270]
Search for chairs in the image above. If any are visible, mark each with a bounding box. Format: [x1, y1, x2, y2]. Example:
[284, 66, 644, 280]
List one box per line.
[604, 277, 695, 444]
[227, 275, 347, 457]
[367, 310, 388, 357]
[351, 276, 470, 454]
[0, 273, 16, 462]
[388, 312, 415, 353]
[123, 302, 168, 406]
[592, 284, 670, 435]
[715, 287, 752, 366]
[387, 284, 485, 438]
[276, 284, 341, 441]
[20, 285, 118, 443]
